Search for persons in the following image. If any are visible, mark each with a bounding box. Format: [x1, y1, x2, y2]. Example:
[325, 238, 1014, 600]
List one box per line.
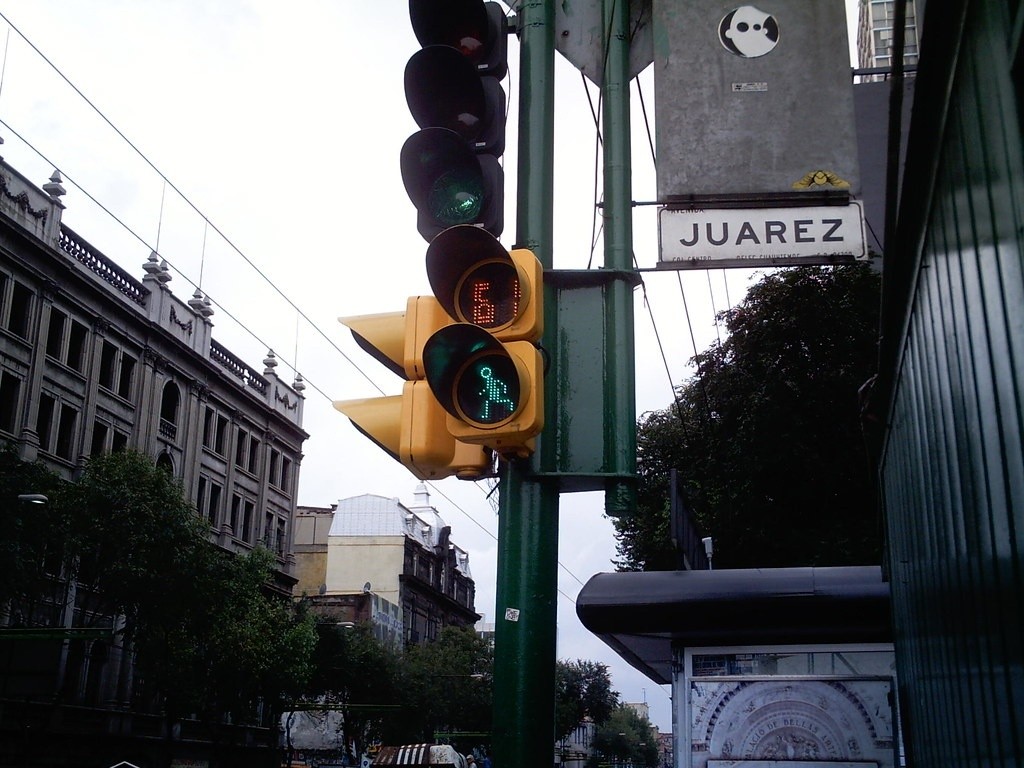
[467, 754, 477, 768]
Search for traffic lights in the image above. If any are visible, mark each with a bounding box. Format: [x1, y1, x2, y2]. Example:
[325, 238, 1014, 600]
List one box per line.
[422, 225, 547, 441]
[400, 1, 507, 244]
[333, 294, 441, 480]
